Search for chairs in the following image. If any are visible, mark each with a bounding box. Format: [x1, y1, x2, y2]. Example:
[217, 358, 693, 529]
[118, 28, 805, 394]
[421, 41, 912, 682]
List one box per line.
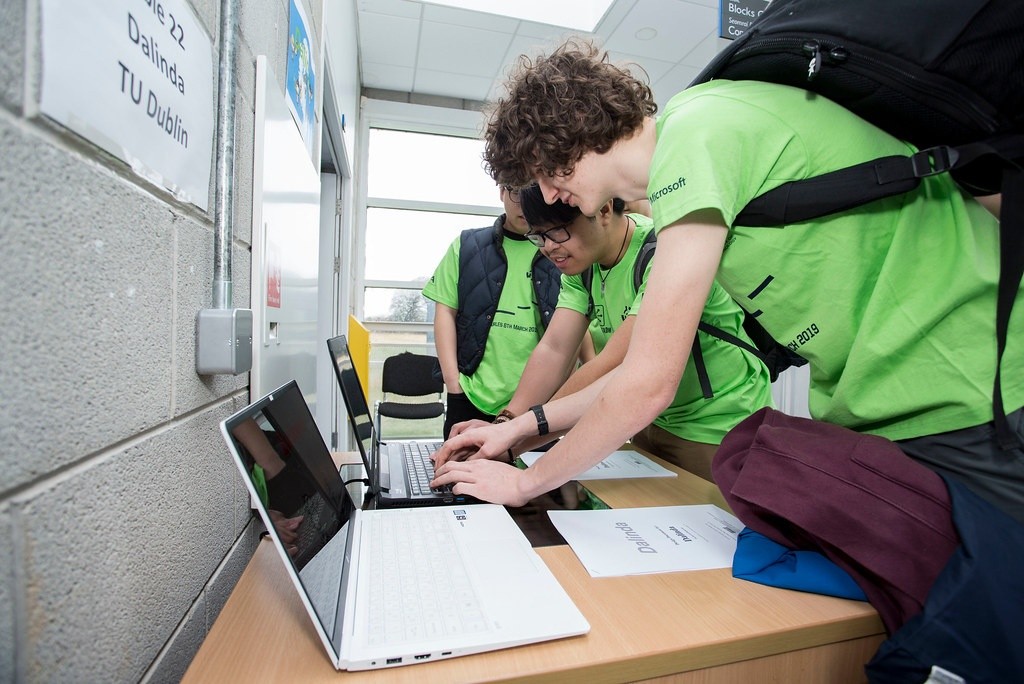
[373, 351, 447, 440]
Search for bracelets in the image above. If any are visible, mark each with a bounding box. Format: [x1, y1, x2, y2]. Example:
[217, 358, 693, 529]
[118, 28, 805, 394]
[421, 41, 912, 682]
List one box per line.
[508, 449, 517, 466]
[492, 410, 516, 424]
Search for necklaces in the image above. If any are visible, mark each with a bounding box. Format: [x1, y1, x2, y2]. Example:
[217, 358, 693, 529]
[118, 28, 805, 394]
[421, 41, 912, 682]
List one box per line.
[597, 214, 630, 293]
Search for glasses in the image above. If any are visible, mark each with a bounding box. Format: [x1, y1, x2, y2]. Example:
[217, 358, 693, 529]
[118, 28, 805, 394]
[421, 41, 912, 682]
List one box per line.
[525, 214, 580, 248]
[503, 184, 521, 204]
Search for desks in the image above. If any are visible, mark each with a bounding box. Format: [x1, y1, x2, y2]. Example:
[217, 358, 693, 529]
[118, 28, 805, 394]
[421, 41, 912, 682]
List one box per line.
[181, 453, 888, 684]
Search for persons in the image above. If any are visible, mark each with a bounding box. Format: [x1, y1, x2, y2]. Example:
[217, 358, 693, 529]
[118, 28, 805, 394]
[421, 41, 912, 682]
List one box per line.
[422, 45, 1024, 527]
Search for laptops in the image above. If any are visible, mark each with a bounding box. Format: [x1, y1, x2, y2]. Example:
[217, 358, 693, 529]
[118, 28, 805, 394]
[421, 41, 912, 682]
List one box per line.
[219, 379, 591, 670]
[326, 334, 492, 508]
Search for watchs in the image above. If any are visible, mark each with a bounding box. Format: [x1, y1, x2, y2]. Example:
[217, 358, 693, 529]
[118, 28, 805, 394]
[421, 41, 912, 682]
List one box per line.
[529, 405, 549, 436]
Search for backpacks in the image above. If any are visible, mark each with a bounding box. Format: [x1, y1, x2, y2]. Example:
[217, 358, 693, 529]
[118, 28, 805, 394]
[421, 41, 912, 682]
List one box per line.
[688, 0, 1024, 451]
[581, 228, 809, 399]
